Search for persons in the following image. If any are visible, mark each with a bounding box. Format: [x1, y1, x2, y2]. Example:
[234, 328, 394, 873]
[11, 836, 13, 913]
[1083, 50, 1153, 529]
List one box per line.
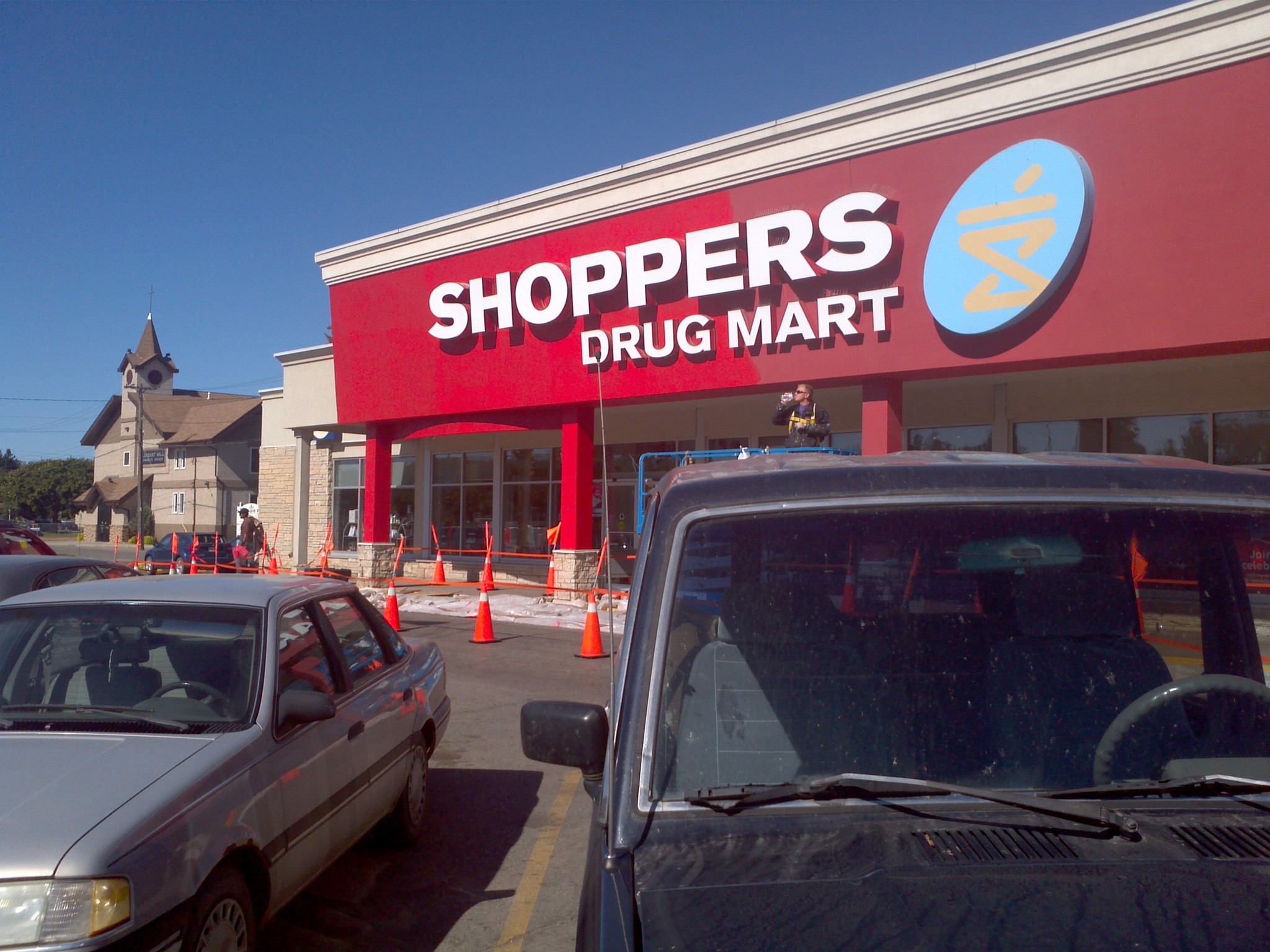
[234, 508, 256, 574]
[771, 384, 830, 448]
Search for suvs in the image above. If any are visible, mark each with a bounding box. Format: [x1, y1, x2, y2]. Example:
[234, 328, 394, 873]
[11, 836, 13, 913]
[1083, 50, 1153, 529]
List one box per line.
[520, 450, 1270, 951]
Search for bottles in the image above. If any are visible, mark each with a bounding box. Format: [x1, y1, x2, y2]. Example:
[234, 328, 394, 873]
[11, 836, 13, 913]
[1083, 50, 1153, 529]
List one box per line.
[390, 513, 400, 525]
[781, 394, 796, 400]
[391, 529, 397, 538]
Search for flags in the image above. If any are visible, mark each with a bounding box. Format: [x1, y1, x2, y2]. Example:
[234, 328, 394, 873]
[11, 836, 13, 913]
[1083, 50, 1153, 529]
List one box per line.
[263, 534, 267, 558]
[115, 536, 119, 554]
[193, 535, 199, 553]
[215, 533, 219, 556]
[172, 532, 179, 555]
[547, 525, 560, 545]
[136, 532, 140, 556]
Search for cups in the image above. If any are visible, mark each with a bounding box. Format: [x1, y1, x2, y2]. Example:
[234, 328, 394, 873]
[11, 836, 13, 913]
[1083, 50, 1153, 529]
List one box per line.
[400, 533, 403, 538]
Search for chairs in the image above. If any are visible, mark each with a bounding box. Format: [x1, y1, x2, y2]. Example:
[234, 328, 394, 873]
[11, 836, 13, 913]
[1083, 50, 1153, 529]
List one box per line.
[674, 581, 925, 791]
[976, 550, 1197, 787]
[166, 638, 253, 719]
[79, 633, 161, 709]
[198, 536, 205, 544]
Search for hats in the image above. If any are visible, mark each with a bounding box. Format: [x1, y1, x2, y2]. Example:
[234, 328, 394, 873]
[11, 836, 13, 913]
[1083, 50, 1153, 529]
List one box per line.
[238, 508, 248, 513]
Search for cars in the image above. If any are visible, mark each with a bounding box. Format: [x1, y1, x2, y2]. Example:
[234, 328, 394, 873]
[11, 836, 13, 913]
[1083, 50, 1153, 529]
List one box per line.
[0, 554, 149, 674]
[0, 526, 58, 557]
[0, 579, 453, 951]
[144, 532, 233, 575]
[60, 522, 78, 531]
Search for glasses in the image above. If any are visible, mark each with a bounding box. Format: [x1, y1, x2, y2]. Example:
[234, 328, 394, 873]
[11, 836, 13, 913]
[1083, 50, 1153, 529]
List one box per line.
[795, 391, 804, 393]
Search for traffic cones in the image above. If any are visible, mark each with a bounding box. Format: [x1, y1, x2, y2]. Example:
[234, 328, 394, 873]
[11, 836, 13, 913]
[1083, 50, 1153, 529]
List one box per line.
[188, 556, 197, 574]
[382, 582, 401, 632]
[573, 594, 609, 659]
[836, 569, 862, 617]
[542, 554, 555, 598]
[168, 565, 176, 575]
[268, 558, 279, 574]
[430, 551, 449, 585]
[1136, 578, 1146, 639]
[132, 561, 140, 571]
[468, 584, 502, 643]
[477, 552, 500, 590]
